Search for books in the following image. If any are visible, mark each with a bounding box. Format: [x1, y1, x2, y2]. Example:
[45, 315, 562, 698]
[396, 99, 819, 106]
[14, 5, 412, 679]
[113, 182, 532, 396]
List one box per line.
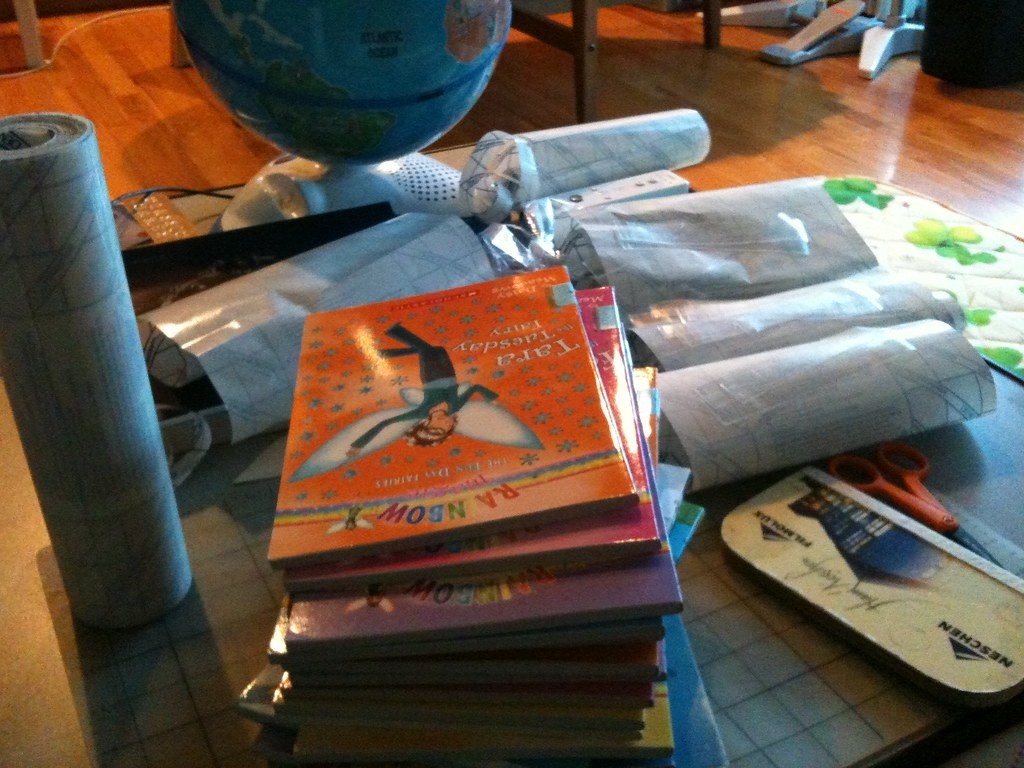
[234, 264, 732, 768]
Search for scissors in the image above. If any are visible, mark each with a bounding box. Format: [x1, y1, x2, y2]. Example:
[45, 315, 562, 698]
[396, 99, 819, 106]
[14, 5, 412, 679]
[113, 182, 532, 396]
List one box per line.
[829, 439, 1009, 571]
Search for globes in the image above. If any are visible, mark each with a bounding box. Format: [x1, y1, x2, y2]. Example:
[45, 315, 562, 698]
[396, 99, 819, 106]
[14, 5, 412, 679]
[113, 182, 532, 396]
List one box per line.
[165, 0, 519, 240]
